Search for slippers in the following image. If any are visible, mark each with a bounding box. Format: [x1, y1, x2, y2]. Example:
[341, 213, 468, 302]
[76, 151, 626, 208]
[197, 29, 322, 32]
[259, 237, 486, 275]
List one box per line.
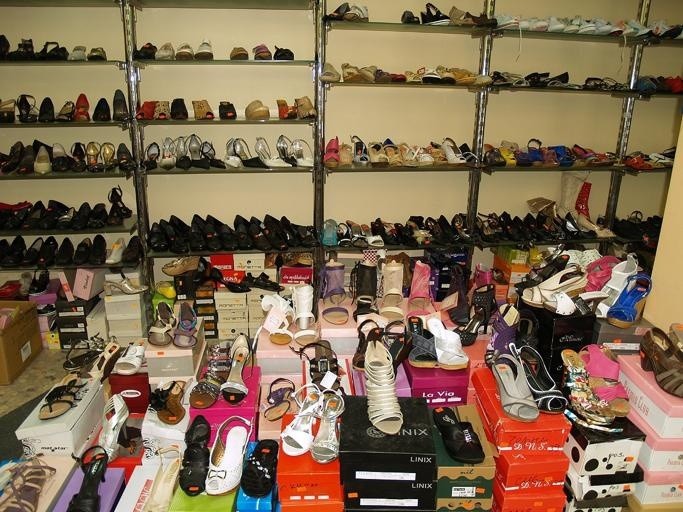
[240, 439, 279, 498]
[432, 406, 485, 463]
[38, 370, 88, 420]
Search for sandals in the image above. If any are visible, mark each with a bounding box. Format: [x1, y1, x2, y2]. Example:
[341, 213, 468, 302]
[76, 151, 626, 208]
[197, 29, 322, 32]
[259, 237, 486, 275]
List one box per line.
[178, 415, 210, 496]
[289, 340, 340, 393]
[492, 354, 540, 423]
[0, 94, 89, 123]
[189, 343, 232, 409]
[280, 384, 324, 456]
[309, 389, 344, 464]
[509, 342, 568, 414]
[141, 444, 182, 511]
[0, 457, 56, 512]
[579, 344, 630, 417]
[63, 301, 198, 375]
[205, 416, 255, 496]
[321, 258, 348, 325]
[484, 303, 520, 370]
[219, 101, 236, 118]
[135, 99, 188, 121]
[261, 294, 295, 345]
[291, 288, 318, 345]
[148, 378, 193, 425]
[277, 96, 317, 119]
[264, 378, 294, 421]
[192, 99, 215, 120]
[245, 101, 269, 120]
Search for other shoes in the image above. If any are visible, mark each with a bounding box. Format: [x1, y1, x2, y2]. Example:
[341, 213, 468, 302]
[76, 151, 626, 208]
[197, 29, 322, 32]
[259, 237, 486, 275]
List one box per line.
[321, 62, 682, 93]
[323, 211, 663, 247]
[666, 322, 682, 351]
[326, 136, 675, 170]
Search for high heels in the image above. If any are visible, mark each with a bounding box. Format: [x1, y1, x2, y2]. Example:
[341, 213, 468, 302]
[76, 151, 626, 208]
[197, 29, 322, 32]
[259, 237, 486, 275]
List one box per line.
[93, 393, 130, 464]
[103, 277, 149, 296]
[351, 250, 498, 380]
[639, 328, 682, 399]
[66, 445, 108, 512]
[363, 340, 404, 434]
[221, 332, 253, 406]
[561, 349, 616, 422]
[513, 248, 652, 329]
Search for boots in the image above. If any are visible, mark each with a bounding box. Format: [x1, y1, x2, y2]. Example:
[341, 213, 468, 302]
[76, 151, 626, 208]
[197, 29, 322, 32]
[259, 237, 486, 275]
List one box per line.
[527, 170, 592, 222]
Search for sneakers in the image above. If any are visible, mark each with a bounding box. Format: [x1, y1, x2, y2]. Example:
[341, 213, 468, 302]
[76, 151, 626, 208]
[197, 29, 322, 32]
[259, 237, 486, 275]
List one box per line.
[37, 303, 57, 331]
[401, 3, 683, 43]
[0, 268, 50, 298]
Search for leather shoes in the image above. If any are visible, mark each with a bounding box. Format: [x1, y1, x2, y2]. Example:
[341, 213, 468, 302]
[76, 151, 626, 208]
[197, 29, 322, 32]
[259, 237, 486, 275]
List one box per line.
[253, 45, 272, 59]
[230, 47, 248, 60]
[93, 98, 110, 121]
[149, 214, 320, 254]
[0, 185, 132, 231]
[274, 45, 294, 60]
[154, 252, 313, 299]
[113, 89, 128, 121]
[0, 139, 137, 176]
[0, 34, 107, 61]
[0, 235, 142, 268]
[133, 39, 213, 60]
[141, 134, 315, 170]
[323, 2, 369, 23]
